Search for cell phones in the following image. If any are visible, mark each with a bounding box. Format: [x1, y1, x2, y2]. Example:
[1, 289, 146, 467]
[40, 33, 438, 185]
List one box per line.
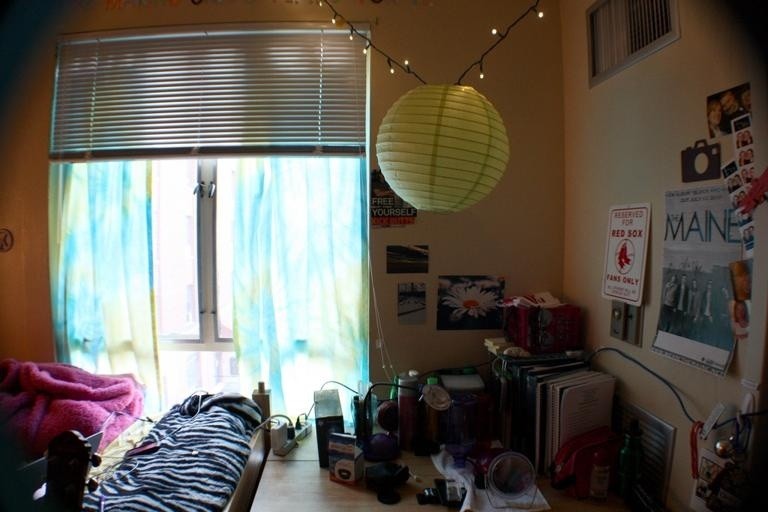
[125, 440, 160, 457]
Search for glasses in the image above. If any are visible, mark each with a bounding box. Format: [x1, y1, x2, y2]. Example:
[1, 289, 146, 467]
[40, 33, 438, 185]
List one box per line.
[538, 310, 554, 350]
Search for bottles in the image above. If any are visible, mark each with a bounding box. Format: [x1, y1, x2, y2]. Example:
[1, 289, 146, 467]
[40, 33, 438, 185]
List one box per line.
[615, 418, 644, 501]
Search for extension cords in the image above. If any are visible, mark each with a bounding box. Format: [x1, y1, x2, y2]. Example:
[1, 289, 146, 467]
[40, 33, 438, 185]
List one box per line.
[273, 420, 312, 456]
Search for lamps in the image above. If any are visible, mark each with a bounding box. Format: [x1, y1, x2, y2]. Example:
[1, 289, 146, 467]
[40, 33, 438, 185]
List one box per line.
[317, 1, 547, 216]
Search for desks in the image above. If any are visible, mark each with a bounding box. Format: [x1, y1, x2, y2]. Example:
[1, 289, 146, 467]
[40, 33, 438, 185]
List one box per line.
[248, 426, 634, 511]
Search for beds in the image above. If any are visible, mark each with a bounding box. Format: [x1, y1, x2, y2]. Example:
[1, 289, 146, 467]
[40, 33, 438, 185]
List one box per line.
[0, 357, 271, 511]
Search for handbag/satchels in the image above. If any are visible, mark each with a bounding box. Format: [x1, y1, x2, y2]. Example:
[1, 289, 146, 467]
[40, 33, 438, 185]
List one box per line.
[552, 426, 624, 498]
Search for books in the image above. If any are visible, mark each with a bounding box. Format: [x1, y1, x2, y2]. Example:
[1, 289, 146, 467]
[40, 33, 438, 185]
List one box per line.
[499, 354, 618, 480]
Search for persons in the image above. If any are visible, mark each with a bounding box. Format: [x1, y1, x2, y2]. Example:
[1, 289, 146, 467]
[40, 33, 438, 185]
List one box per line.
[705, 83, 756, 252]
[658, 263, 752, 352]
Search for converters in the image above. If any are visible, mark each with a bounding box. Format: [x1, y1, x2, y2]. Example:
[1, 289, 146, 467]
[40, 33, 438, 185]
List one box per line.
[271, 421, 287, 450]
[287, 423, 295, 440]
